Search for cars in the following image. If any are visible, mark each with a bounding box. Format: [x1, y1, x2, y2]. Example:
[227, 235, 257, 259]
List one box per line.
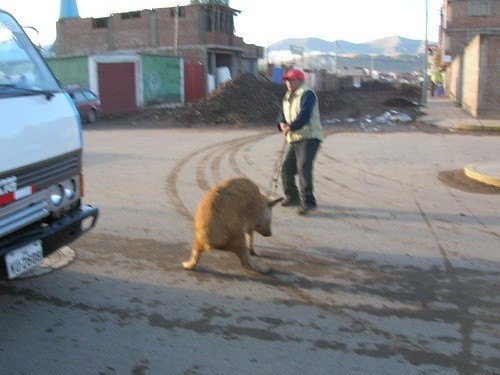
[64, 88, 102, 123]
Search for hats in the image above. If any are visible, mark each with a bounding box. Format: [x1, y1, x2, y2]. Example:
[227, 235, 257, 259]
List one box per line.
[282, 69, 304, 81]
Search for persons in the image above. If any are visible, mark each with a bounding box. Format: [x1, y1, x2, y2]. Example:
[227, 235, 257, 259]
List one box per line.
[429, 72, 445, 97]
[276, 69, 324, 216]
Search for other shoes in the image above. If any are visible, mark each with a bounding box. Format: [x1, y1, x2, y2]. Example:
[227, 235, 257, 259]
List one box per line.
[281, 197, 299, 206]
[297, 204, 317, 215]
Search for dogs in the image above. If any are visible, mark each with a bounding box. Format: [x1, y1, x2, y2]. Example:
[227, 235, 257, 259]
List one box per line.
[181, 177, 284, 275]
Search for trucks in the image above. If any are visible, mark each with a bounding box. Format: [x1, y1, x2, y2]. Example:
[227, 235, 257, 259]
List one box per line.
[0, 10, 99, 291]
